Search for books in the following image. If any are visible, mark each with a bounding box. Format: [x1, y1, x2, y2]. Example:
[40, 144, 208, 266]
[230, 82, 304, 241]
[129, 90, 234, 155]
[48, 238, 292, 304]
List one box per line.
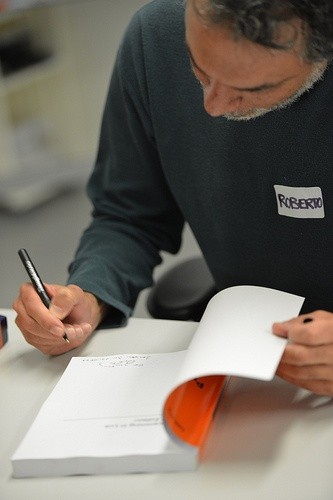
[9, 284, 306, 479]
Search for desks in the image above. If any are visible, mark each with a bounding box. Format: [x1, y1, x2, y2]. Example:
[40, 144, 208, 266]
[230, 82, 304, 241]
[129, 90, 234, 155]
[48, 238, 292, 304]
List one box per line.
[0, 309, 333, 500]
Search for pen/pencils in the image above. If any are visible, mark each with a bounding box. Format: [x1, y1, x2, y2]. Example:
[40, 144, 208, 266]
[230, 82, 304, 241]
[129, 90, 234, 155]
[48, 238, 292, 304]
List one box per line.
[18, 248, 70, 344]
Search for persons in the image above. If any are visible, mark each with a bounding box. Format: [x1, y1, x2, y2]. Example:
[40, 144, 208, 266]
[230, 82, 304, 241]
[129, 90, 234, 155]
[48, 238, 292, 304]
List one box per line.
[13, 1, 333, 401]
